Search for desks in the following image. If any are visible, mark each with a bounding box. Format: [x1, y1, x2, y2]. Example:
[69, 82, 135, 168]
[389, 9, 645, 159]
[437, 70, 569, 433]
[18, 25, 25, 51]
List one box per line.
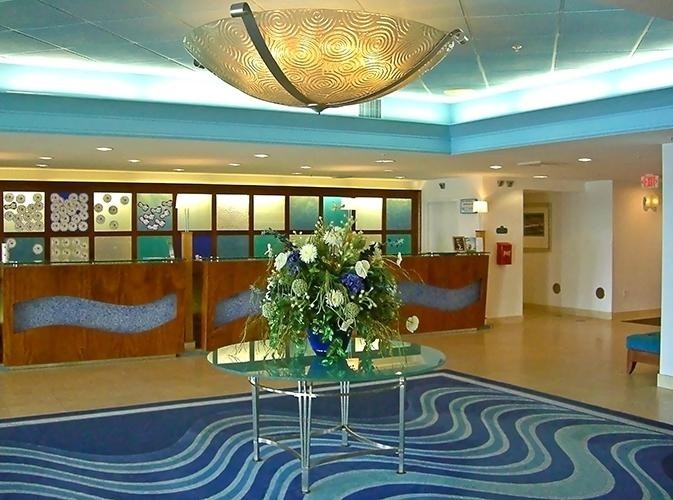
[206, 337, 448, 496]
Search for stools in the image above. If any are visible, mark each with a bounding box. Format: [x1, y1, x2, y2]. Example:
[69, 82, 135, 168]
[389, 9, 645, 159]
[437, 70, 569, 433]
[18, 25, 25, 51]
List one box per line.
[624, 331, 660, 374]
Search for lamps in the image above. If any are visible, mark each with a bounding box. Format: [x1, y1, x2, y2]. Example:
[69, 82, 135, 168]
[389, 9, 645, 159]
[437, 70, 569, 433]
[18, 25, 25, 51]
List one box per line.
[643, 193, 660, 211]
[473, 201, 488, 213]
[180, 2, 469, 116]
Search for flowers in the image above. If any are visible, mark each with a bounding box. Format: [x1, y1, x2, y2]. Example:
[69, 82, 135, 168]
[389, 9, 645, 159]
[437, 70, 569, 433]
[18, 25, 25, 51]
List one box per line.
[232, 213, 426, 377]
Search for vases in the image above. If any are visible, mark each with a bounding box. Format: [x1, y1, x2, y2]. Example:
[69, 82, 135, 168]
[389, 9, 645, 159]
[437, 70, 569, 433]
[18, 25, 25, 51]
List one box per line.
[306, 325, 352, 358]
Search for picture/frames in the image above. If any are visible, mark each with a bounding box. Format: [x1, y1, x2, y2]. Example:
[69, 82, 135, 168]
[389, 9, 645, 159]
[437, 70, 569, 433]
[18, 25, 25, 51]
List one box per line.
[452, 236, 465, 252]
[523, 203, 552, 253]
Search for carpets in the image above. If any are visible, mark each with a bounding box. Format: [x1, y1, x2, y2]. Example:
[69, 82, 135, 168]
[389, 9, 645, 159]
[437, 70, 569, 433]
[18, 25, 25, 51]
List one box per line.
[621, 317, 660, 326]
[0, 370, 672, 500]
